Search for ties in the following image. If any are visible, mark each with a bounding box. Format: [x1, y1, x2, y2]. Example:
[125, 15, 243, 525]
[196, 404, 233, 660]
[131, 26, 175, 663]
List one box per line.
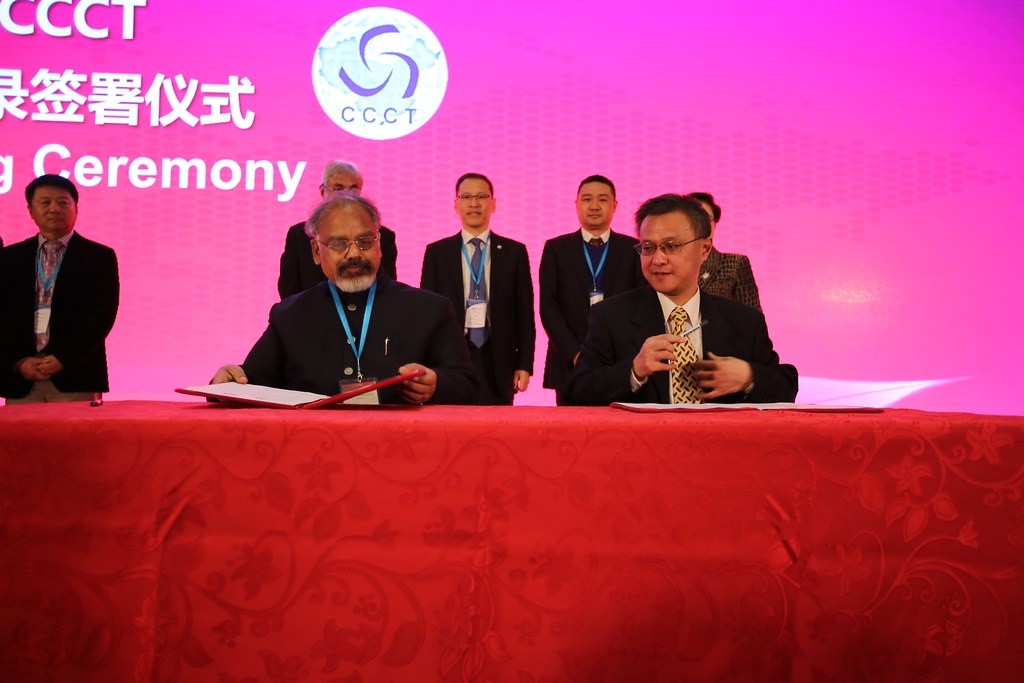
[467, 238, 486, 348]
[590, 238, 602, 246]
[670, 306, 703, 403]
[36, 241, 64, 351]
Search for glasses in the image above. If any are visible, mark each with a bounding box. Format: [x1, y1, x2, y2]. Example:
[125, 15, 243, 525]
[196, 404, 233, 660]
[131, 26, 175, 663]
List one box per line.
[314, 232, 379, 251]
[455, 192, 492, 203]
[634, 235, 707, 255]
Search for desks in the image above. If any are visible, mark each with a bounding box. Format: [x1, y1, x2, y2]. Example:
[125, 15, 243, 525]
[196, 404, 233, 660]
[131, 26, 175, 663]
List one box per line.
[0, 400, 1024, 683]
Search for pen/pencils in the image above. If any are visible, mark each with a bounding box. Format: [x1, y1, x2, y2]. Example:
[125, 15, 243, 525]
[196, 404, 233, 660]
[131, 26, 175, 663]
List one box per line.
[670, 319, 709, 345]
[384, 336, 390, 355]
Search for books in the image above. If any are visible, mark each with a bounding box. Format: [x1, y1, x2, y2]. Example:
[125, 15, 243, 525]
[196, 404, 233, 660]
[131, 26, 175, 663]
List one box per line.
[610, 402, 884, 414]
[175, 370, 424, 409]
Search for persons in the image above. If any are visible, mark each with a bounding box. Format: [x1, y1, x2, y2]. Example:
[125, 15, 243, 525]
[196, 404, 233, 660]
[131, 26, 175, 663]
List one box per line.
[539, 175, 645, 406]
[277, 158, 397, 299]
[420, 173, 537, 407]
[690, 191, 763, 311]
[570, 193, 799, 405]
[205, 192, 481, 406]
[0, 175, 119, 405]
